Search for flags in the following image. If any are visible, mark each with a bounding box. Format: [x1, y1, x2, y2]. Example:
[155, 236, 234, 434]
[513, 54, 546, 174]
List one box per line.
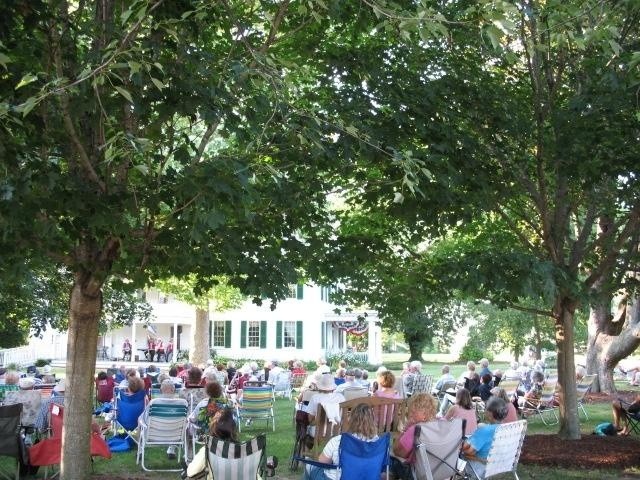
[147, 322, 157, 336]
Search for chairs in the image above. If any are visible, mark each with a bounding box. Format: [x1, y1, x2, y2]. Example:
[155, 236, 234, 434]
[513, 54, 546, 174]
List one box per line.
[1, 368, 111, 479]
[390, 418, 469, 479]
[135, 397, 190, 473]
[293, 431, 391, 479]
[306, 401, 339, 458]
[109, 367, 599, 433]
[457, 419, 527, 479]
[183, 398, 243, 465]
[618, 396, 639, 438]
[202, 433, 279, 479]
[112, 384, 149, 444]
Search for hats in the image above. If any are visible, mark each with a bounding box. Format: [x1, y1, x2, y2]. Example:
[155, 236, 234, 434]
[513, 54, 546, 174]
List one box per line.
[44, 364, 51, 373]
[27, 366, 35, 373]
[316, 374, 337, 391]
[20, 378, 35, 388]
[478, 358, 489, 366]
[346, 371, 354, 376]
[312, 371, 322, 383]
[53, 378, 65, 391]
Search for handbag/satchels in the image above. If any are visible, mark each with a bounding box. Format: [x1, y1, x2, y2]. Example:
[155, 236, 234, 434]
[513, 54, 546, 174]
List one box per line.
[108, 434, 134, 452]
[595, 422, 614, 436]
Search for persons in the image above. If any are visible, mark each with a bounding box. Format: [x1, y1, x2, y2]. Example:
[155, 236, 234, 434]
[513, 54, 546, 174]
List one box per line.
[303, 403, 380, 479]
[0, 371, 61, 428]
[97, 359, 306, 460]
[185, 413, 262, 480]
[626, 365, 640, 386]
[123, 339, 131, 360]
[458, 397, 509, 480]
[611, 395, 640, 435]
[381, 394, 455, 479]
[298, 358, 545, 449]
[144, 337, 173, 362]
